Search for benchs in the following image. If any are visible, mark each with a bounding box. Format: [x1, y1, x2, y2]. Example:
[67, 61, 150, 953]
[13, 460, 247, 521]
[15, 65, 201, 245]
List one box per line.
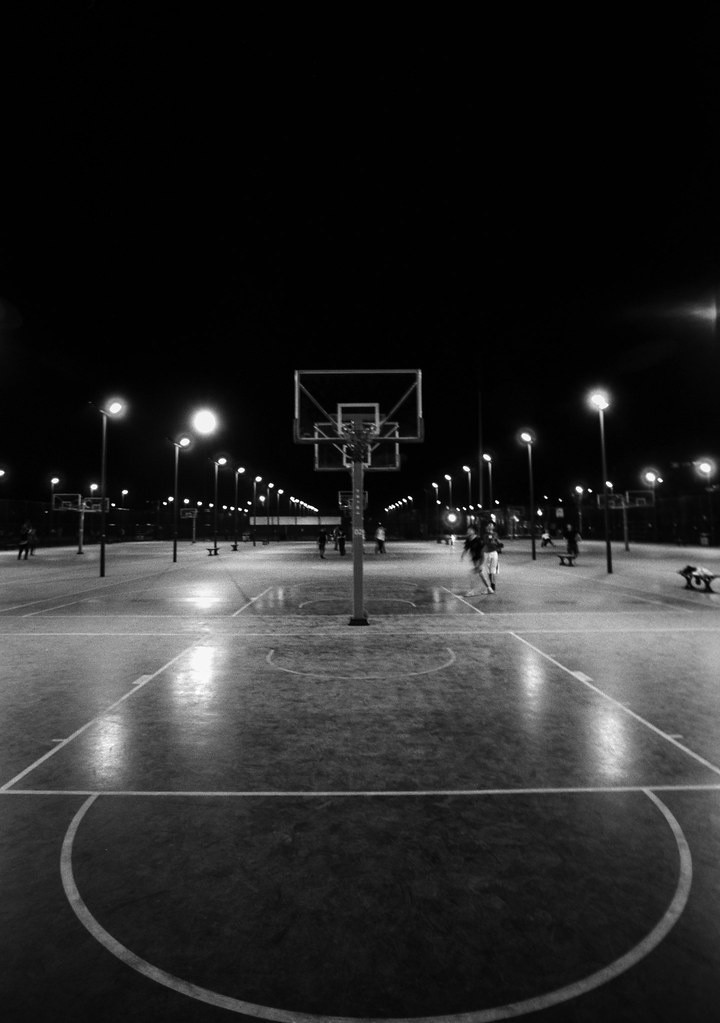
[556, 553, 577, 567]
[206, 547, 220, 556]
[230, 544, 239, 551]
[677, 569, 720, 594]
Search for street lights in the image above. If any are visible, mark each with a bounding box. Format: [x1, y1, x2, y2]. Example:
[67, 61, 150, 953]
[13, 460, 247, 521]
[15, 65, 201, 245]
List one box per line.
[645, 471, 658, 509]
[234, 467, 245, 545]
[592, 394, 613, 574]
[213, 458, 226, 555]
[277, 489, 285, 542]
[520, 432, 537, 561]
[698, 462, 710, 488]
[461, 465, 472, 505]
[432, 481, 441, 543]
[266, 482, 275, 544]
[100, 401, 123, 578]
[121, 489, 129, 505]
[576, 484, 585, 535]
[289, 496, 319, 541]
[50, 477, 59, 501]
[172, 436, 192, 562]
[252, 476, 263, 547]
[90, 483, 100, 498]
[445, 474, 454, 546]
[482, 454, 492, 512]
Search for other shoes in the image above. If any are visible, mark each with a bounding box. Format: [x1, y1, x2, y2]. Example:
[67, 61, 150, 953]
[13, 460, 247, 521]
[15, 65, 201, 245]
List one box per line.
[465, 590, 475, 596]
[487, 585, 495, 594]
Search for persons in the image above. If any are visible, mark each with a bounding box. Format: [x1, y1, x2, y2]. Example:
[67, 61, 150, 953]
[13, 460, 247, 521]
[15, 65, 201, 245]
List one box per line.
[17, 523, 35, 560]
[374, 526, 387, 553]
[460, 525, 495, 597]
[333, 525, 346, 556]
[562, 525, 582, 560]
[541, 531, 554, 547]
[318, 528, 327, 559]
[483, 523, 504, 591]
